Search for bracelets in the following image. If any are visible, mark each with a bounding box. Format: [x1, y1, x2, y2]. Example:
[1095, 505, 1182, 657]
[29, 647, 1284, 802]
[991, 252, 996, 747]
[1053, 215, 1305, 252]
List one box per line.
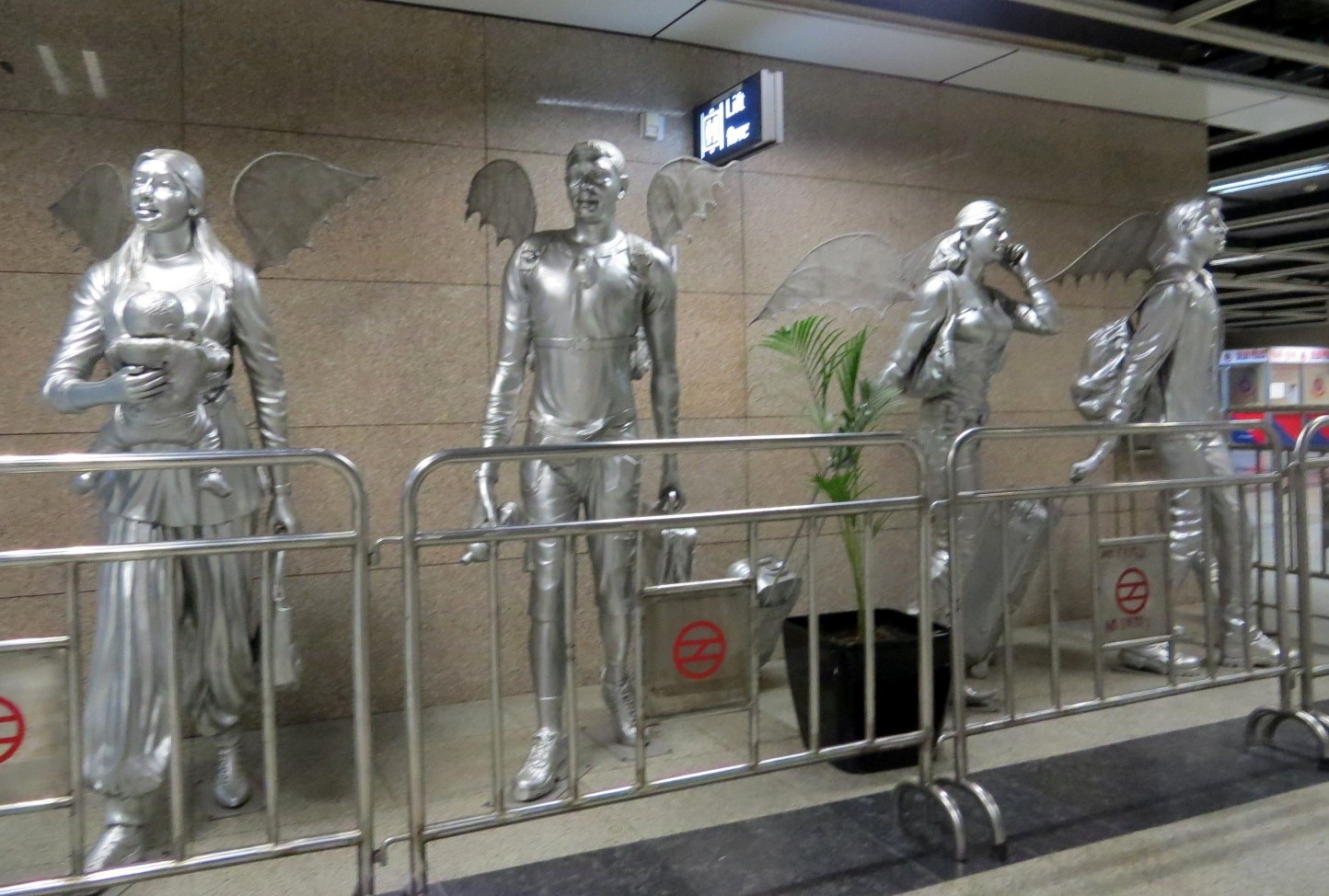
[1021, 271, 1037, 287]
[271, 483, 293, 495]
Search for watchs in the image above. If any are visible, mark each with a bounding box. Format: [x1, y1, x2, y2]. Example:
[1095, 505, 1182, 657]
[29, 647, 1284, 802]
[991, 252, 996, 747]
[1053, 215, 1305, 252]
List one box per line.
[472, 468, 498, 482]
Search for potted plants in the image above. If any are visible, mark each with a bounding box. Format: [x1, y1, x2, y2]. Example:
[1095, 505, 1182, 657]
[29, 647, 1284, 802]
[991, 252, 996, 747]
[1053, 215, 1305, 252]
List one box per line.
[748, 314, 956, 774]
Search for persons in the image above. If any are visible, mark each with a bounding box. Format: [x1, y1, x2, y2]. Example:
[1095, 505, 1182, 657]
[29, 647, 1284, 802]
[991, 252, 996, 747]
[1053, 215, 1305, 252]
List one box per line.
[1071, 195, 1302, 677]
[42, 148, 301, 896]
[467, 139, 688, 803]
[879, 199, 1062, 703]
